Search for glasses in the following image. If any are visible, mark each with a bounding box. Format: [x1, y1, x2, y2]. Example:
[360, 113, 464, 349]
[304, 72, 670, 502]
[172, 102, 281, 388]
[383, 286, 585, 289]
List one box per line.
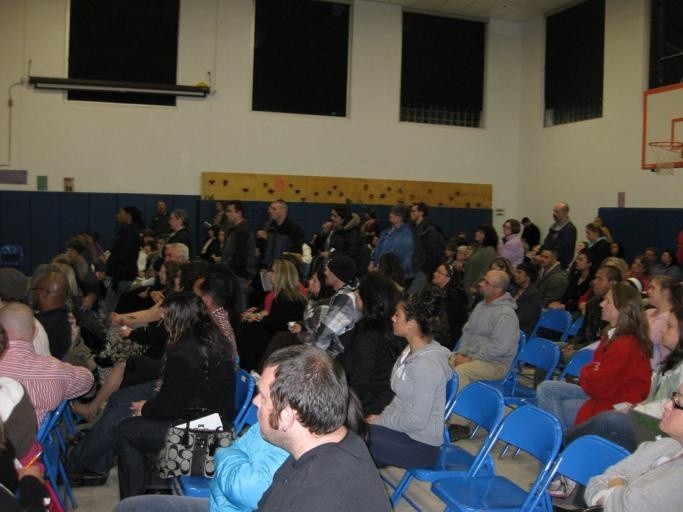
[669, 390, 683, 411]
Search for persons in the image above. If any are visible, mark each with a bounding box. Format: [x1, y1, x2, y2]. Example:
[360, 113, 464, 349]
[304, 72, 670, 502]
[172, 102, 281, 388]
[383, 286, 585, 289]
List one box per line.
[0, 198, 682, 512]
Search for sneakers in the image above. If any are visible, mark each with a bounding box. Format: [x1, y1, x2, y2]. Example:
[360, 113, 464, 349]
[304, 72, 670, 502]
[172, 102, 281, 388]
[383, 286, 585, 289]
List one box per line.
[51, 465, 110, 487]
[446, 423, 472, 444]
[547, 476, 569, 498]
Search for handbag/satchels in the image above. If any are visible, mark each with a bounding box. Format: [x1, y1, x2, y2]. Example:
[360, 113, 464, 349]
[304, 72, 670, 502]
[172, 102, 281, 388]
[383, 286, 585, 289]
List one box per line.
[154, 407, 237, 481]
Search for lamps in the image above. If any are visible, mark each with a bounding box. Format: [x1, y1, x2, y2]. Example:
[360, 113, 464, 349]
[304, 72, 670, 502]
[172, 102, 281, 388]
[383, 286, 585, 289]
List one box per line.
[24, 58, 212, 99]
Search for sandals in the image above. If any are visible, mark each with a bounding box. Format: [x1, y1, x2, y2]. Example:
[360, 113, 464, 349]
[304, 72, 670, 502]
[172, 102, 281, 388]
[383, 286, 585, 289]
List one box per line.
[70, 402, 98, 420]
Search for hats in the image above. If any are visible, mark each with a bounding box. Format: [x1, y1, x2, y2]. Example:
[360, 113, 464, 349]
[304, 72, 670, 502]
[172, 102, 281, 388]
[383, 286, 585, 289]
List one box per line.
[627, 277, 643, 292]
[328, 254, 357, 282]
